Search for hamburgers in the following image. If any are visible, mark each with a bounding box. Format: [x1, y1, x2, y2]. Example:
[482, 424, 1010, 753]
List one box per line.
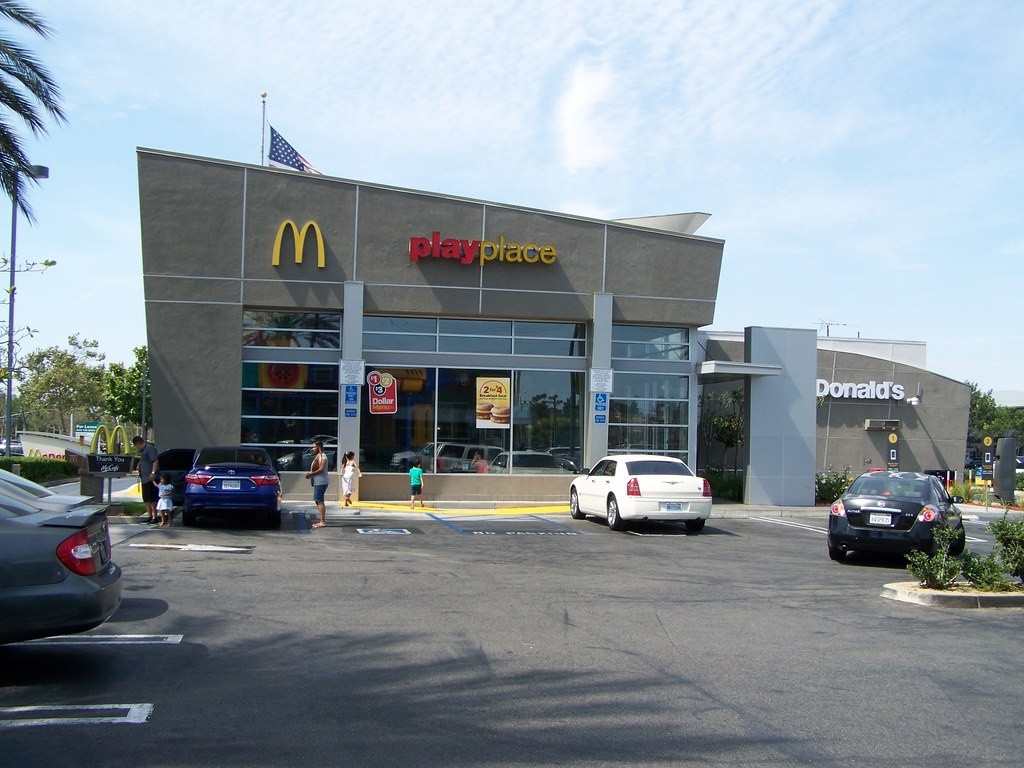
[476, 402, 494, 420]
[490, 406, 511, 423]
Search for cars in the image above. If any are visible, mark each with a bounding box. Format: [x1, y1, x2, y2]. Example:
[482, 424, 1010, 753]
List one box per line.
[390, 442, 440, 473]
[277, 435, 367, 469]
[825, 468, 966, 560]
[568, 454, 713, 533]
[487, 447, 582, 475]
[0, 467, 123, 647]
[156, 446, 283, 529]
[544, 447, 581, 462]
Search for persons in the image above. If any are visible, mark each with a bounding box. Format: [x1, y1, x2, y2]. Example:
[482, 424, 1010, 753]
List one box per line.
[132, 436, 175, 525]
[437, 449, 457, 473]
[340, 449, 360, 507]
[305, 441, 328, 528]
[470, 450, 488, 473]
[409, 459, 424, 510]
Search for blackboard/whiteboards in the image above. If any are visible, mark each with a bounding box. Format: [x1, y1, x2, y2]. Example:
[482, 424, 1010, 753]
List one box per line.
[85, 453, 133, 478]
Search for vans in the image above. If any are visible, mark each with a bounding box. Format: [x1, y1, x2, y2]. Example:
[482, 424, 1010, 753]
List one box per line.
[429, 442, 503, 473]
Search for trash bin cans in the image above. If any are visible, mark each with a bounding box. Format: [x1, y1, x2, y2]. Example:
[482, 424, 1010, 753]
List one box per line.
[77, 466, 105, 502]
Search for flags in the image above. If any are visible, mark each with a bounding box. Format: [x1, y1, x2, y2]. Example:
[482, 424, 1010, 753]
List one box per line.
[269, 125, 327, 176]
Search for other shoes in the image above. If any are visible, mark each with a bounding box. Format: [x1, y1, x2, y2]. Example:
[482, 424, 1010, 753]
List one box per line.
[141, 517, 158, 524]
[312, 522, 326, 527]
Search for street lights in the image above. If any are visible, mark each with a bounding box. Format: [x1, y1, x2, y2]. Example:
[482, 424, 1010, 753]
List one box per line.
[5, 163, 51, 459]
[135, 364, 147, 443]
